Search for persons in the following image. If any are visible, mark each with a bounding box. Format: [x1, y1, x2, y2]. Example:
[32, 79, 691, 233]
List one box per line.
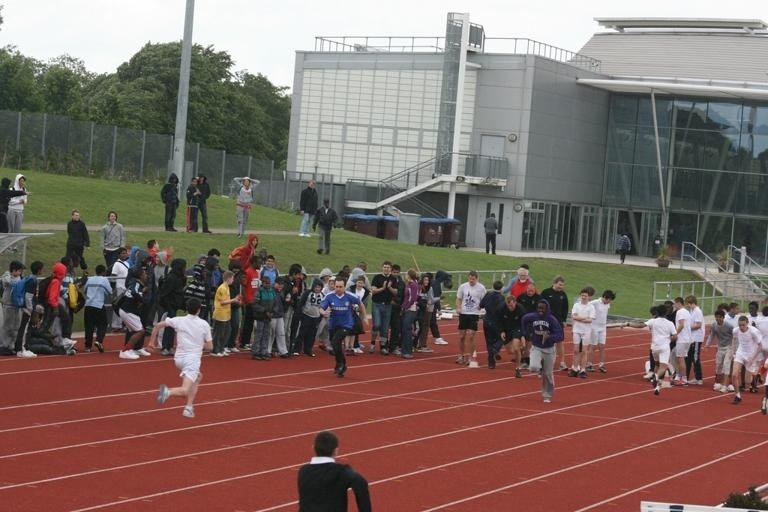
[6, 173, 28, 233]
[190, 174, 212, 233]
[65, 209, 91, 277]
[484, 213, 499, 255]
[298, 179, 319, 238]
[481, 279, 505, 371]
[0, 172, 34, 233]
[99, 210, 125, 268]
[316, 196, 339, 256]
[161, 172, 181, 232]
[626, 295, 740, 404]
[297, 430, 373, 512]
[454, 268, 486, 363]
[293, 260, 452, 377]
[505, 263, 616, 403]
[186, 177, 199, 233]
[232, 176, 261, 239]
[111, 247, 149, 360]
[1, 255, 115, 356]
[615, 231, 632, 265]
[144, 236, 300, 418]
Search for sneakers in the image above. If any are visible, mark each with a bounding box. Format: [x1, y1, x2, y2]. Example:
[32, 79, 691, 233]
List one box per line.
[260, 354, 271, 361]
[733, 394, 742, 403]
[537, 368, 542, 379]
[138, 349, 152, 356]
[434, 338, 449, 345]
[94, 341, 104, 352]
[586, 363, 596, 372]
[381, 349, 389, 355]
[83, 344, 92, 353]
[279, 353, 289, 357]
[222, 351, 229, 356]
[712, 384, 721, 389]
[368, 346, 378, 353]
[252, 354, 261, 360]
[119, 350, 140, 360]
[417, 347, 435, 353]
[170, 348, 175, 355]
[293, 352, 302, 356]
[579, 370, 587, 379]
[335, 364, 347, 376]
[469, 359, 480, 369]
[160, 351, 170, 355]
[719, 387, 727, 394]
[16, 350, 37, 358]
[688, 378, 704, 385]
[456, 355, 466, 364]
[515, 368, 522, 378]
[560, 362, 568, 371]
[599, 365, 607, 374]
[210, 351, 224, 357]
[544, 397, 553, 406]
[393, 347, 402, 355]
[568, 367, 578, 377]
[225, 347, 231, 353]
[158, 384, 169, 404]
[231, 347, 240, 352]
[353, 347, 364, 354]
[183, 406, 196, 418]
[308, 351, 316, 358]
[643, 370, 655, 379]
[404, 354, 413, 359]
